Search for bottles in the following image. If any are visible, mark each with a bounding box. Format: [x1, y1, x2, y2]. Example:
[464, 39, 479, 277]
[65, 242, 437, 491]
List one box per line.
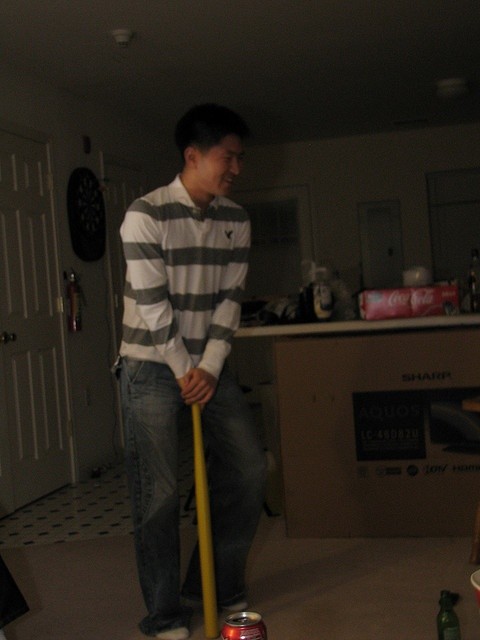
[437, 590, 461, 640]
[468, 248, 480, 313]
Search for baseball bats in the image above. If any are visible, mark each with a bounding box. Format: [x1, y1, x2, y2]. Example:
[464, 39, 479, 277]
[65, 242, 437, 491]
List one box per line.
[192, 403, 218, 639]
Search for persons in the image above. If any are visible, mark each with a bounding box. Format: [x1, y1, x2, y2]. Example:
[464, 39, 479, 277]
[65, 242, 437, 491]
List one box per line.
[110, 103, 267, 640]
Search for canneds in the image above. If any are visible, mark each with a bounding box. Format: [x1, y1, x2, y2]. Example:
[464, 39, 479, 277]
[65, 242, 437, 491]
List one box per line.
[220, 609, 268, 639]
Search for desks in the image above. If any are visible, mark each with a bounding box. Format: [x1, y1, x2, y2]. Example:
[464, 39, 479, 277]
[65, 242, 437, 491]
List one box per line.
[231, 314, 479, 507]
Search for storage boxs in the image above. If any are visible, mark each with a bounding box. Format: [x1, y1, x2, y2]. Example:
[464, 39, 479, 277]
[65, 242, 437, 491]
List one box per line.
[276, 325, 480, 537]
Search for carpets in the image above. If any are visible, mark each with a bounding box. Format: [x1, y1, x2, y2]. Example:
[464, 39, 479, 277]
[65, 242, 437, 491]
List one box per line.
[0, 518, 480, 640]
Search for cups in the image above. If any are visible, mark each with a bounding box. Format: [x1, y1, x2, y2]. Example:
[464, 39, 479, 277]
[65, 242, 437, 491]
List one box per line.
[312, 282, 334, 322]
